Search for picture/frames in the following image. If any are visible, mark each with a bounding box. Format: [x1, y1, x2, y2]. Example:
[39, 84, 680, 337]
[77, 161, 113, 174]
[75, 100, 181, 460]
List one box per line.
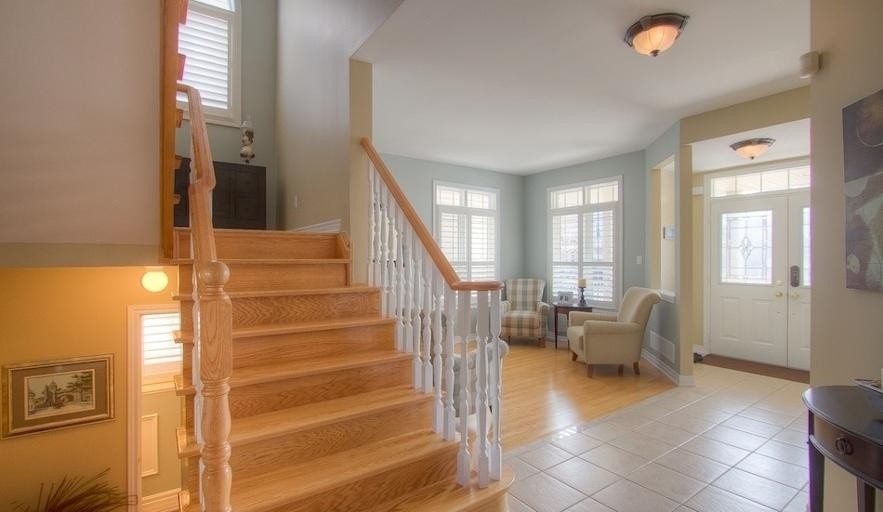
[140, 414, 159, 479]
[0, 355, 115, 436]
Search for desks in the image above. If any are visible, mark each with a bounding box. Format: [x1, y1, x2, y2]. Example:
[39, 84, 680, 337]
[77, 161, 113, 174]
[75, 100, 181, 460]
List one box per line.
[801, 385, 883, 512]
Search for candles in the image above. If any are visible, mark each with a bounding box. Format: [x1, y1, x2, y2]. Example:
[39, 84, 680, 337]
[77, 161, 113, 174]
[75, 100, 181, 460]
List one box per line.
[578, 279, 586, 287]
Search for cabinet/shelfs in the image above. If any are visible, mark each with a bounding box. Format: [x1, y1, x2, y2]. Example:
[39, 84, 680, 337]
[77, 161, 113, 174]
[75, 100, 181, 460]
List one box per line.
[174, 154, 267, 229]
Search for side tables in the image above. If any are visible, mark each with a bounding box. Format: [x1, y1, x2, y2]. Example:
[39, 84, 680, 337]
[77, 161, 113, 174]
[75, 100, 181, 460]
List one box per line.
[554, 304, 593, 349]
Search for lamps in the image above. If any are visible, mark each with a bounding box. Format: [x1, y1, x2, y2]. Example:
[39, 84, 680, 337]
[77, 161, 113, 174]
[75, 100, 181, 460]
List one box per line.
[142, 270, 170, 294]
[623, 13, 688, 58]
[726, 137, 774, 162]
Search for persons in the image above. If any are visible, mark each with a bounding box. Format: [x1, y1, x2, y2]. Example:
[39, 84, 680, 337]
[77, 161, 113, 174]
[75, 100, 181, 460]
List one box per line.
[847, 214, 874, 288]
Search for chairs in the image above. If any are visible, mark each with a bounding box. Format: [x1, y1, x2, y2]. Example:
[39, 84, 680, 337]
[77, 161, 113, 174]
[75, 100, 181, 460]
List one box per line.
[500, 278, 550, 349]
[392, 305, 509, 420]
[567, 286, 662, 381]
